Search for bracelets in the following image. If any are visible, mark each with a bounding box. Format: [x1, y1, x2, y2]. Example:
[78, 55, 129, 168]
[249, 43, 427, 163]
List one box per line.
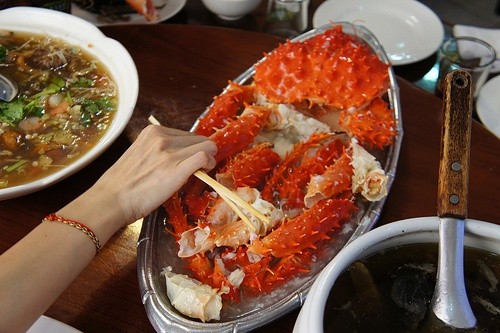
[43, 215, 102, 256]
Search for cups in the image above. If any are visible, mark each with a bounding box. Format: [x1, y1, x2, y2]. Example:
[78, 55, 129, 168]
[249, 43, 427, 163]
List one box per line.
[435, 37, 500, 107]
[266, 0, 310, 38]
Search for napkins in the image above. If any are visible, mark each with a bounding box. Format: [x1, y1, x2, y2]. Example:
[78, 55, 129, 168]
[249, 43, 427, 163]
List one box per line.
[453, 25, 500, 73]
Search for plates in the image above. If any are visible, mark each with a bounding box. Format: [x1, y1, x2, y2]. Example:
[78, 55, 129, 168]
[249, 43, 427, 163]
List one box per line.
[476, 74, 500, 144]
[313, 0, 445, 66]
[137, 21, 403, 333]
[70, 0, 187, 26]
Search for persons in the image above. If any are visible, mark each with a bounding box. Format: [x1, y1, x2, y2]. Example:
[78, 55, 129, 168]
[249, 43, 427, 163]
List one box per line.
[0, 124, 218, 333]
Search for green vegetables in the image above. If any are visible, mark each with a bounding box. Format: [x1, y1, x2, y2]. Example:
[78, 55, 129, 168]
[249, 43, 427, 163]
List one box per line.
[0, 44, 112, 173]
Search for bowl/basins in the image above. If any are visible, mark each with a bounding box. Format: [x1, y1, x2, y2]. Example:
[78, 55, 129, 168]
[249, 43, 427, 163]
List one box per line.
[201, 0, 263, 22]
[0, 6, 139, 203]
[292, 217, 497, 333]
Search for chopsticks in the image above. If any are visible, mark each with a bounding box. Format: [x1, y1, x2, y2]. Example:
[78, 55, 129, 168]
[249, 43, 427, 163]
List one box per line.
[148, 115, 270, 232]
